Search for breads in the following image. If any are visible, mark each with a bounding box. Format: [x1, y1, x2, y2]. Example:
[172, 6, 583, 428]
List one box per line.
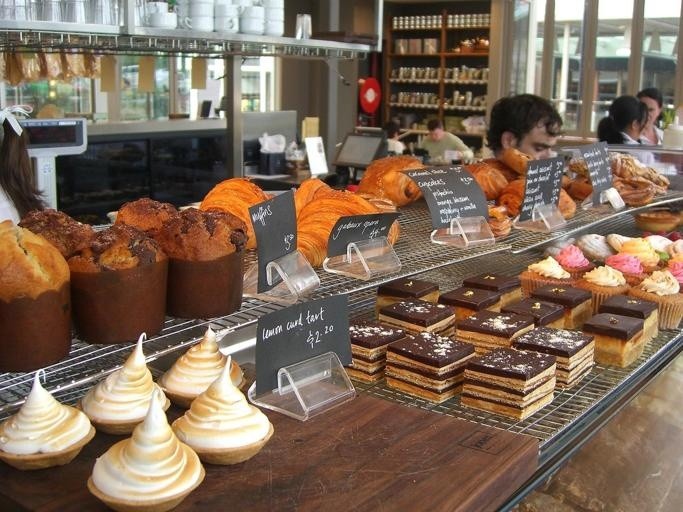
[569, 150, 669, 204]
[361, 157, 425, 206]
[458, 147, 593, 236]
[200, 178, 400, 268]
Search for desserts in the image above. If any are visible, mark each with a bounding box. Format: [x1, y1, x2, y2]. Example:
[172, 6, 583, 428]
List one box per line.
[0, 325, 275, 511]
[1, 196, 248, 372]
[345, 271, 661, 421]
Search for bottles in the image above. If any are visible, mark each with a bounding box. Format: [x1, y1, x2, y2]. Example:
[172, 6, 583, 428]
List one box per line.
[398, 65, 488, 80]
[662, 115, 683, 150]
[397, 89, 472, 107]
[392, 12, 491, 29]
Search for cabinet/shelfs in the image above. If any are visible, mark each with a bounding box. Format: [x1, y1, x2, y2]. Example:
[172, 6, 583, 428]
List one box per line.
[381, 1, 530, 134]
[0, 151, 681, 512]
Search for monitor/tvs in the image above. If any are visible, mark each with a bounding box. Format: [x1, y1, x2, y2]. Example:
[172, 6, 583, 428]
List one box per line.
[331, 133, 389, 169]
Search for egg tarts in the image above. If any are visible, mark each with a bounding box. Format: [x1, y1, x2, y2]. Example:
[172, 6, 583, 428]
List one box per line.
[635, 209, 682, 232]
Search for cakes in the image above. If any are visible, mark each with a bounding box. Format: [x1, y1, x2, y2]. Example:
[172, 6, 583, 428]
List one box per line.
[526, 234, 683, 328]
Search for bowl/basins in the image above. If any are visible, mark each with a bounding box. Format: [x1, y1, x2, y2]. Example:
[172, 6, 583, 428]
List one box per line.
[634, 213, 680, 232]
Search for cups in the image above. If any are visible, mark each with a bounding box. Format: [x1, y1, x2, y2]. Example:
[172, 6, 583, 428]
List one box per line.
[0, 0, 118, 25]
[147, 0, 286, 37]
[256, 150, 286, 175]
[293, 13, 312, 39]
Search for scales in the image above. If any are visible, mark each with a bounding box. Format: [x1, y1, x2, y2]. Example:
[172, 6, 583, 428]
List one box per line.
[17, 118, 114, 232]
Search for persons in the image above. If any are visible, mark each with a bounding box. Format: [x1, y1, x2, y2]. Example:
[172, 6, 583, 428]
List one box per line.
[419, 120, 475, 165]
[0, 104, 46, 226]
[635, 87, 663, 162]
[484, 94, 563, 163]
[383, 121, 408, 156]
[597, 94, 655, 165]
